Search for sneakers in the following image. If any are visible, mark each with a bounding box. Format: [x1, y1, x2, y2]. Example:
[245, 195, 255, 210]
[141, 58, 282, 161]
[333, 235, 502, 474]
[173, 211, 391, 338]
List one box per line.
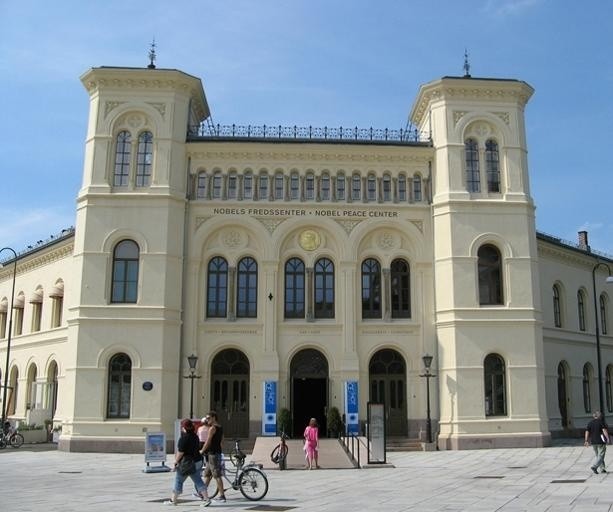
[164, 500, 178, 506]
[200, 499, 211, 508]
[213, 495, 226, 503]
[591, 467, 607, 475]
[193, 492, 202, 500]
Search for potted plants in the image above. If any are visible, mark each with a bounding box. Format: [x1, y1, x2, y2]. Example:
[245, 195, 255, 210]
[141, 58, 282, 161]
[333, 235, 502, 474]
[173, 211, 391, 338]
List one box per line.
[277, 408, 291, 439]
[327, 407, 341, 438]
[16, 422, 47, 443]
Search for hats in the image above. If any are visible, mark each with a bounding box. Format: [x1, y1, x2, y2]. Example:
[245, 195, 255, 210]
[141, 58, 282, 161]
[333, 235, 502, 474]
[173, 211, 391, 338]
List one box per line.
[201, 417, 208, 423]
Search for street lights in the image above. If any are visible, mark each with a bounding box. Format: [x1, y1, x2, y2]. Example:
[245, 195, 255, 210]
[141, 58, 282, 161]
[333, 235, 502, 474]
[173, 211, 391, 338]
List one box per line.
[590, 263, 612, 431]
[183, 352, 200, 420]
[419, 353, 438, 443]
[0, 246, 17, 425]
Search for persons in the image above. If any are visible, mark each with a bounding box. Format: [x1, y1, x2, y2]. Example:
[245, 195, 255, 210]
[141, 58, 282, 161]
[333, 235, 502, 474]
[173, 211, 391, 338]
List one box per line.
[196, 417, 208, 469]
[302, 438, 309, 469]
[163, 419, 211, 507]
[192, 410, 226, 502]
[583, 410, 610, 474]
[303, 417, 320, 469]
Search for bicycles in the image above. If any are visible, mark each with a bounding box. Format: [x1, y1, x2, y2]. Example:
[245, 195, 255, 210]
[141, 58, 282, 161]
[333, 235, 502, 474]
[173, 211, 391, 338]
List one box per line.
[192, 454, 268, 501]
[0, 426, 24, 450]
[271, 432, 290, 464]
[229, 438, 246, 467]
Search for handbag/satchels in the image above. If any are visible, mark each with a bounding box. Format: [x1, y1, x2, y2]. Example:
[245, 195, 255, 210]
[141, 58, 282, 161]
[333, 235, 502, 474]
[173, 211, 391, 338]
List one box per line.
[179, 461, 197, 476]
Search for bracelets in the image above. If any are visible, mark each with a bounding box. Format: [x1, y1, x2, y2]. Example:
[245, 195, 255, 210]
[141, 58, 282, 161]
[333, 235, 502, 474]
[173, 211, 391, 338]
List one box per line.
[585, 440, 588, 442]
[173, 462, 177, 466]
